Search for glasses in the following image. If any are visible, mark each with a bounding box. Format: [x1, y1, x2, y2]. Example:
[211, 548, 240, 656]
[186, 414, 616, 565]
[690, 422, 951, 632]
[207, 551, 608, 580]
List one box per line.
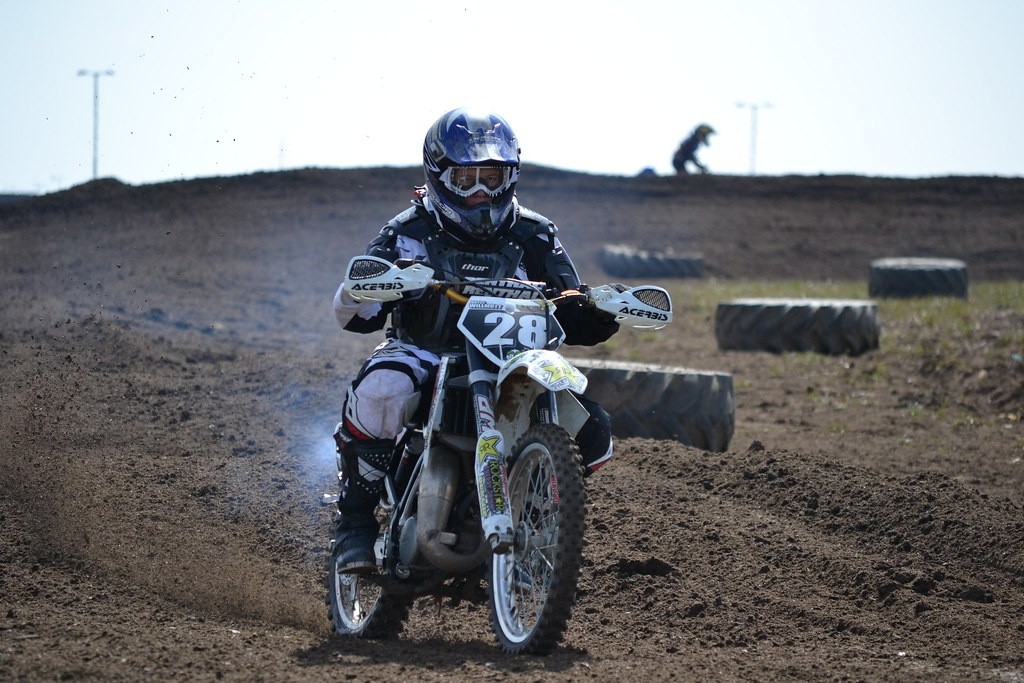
[442, 164, 516, 198]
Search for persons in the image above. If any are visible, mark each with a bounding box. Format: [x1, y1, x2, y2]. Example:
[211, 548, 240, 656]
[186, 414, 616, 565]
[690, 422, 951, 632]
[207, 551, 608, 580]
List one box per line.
[332, 106, 632, 573]
[672, 124, 717, 176]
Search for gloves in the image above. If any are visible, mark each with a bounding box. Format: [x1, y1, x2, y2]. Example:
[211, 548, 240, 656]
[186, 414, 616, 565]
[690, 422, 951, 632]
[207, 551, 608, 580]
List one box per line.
[608, 282, 632, 294]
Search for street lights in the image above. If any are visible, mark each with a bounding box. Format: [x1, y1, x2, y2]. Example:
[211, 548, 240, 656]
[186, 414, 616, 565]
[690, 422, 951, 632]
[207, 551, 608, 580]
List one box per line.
[735, 100, 771, 175]
[78, 68, 119, 179]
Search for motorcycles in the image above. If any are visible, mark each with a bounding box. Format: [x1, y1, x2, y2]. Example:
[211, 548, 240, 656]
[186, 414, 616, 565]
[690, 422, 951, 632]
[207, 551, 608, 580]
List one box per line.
[326, 265, 671, 663]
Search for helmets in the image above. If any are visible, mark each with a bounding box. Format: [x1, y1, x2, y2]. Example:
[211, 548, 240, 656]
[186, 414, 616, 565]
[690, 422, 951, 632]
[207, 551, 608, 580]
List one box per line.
[423, 106, 521, 243]
[696, 125, 714, 145]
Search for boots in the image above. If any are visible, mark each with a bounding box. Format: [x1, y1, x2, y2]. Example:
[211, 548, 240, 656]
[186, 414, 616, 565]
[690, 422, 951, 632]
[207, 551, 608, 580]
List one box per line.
[330, 421, 397, 572]
[515, 471, 549, 593]
